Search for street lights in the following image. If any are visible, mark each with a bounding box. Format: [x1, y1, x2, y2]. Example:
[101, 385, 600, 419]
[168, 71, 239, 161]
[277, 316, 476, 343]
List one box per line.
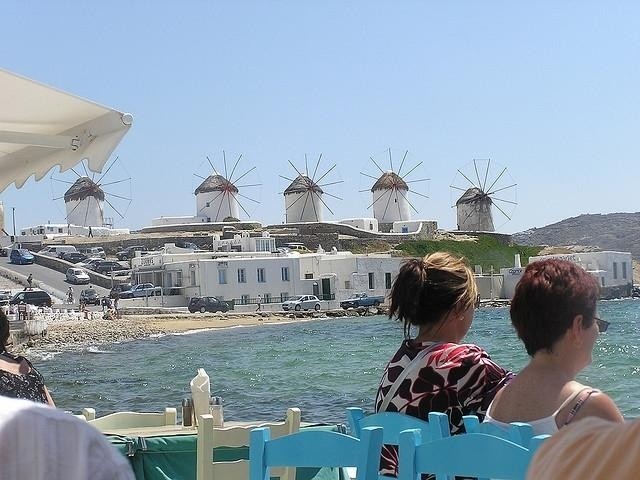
[11, 206, 15, 235]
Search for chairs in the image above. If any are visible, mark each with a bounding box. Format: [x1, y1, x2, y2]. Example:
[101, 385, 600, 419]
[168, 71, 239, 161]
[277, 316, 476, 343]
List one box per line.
[247, 421, 384, 478]
[193, 408, 302, 478]
[390, 424, 552, 480]
[349, 408, 456, 480]
[76, 406, 179, 434]
[462, 414, 535, 447]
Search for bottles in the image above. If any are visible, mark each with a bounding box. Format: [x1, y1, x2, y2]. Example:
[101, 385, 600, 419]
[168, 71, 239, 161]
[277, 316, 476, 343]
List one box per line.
[182, 398, 195, 428]
[209, 396, 224, 427]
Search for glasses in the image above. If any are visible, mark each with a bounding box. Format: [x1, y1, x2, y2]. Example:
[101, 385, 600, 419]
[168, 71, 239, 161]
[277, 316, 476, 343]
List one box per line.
[568, 312, 609, 332]
[450, 293, 480, 309]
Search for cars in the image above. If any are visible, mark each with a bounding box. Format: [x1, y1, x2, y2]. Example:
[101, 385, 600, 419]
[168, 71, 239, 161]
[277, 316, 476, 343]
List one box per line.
[184, 241, 212, 250]
[188, 296, 230, 313]
[119, 282, 162, 297]
[9, 248, 35, 265]
[109, 284, 132, 298]
[0, 294, 9, 306]
[288, 244, 313, 256]
[37, 245, 164, 277]
[65, 268, 91, 284]
[79, 289, 100, 306]
[281, 294, 322, 311]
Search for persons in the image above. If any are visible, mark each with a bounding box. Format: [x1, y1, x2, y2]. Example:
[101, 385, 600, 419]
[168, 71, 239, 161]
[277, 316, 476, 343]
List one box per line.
[481, 259, 626, 438]
[0, 307, 56, 408]
[27, 273, 33, 288]
[67, 223, 72, 235]
[0, 394, 135, 480]
[524, 415, 640, 480]
[373, 251, 517, 480]
[88, 225, 94, 238]
[255, 294, 262, 312]
[65, 285, 119, 320]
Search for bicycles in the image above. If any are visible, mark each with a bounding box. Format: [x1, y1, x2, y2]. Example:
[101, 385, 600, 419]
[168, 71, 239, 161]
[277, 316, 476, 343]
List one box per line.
[66, 293, 74, 304]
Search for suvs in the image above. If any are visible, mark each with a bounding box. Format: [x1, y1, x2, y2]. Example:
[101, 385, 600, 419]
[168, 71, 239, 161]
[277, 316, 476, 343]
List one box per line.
[0, 291, 52, 308]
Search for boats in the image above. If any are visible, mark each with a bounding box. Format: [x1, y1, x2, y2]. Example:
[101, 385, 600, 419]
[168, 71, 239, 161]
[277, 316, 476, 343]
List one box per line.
[632, 283, 640, 300]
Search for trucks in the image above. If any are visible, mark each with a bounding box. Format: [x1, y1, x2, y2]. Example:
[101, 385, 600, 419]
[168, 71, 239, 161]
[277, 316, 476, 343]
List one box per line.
[340, 292, 385, 310]
